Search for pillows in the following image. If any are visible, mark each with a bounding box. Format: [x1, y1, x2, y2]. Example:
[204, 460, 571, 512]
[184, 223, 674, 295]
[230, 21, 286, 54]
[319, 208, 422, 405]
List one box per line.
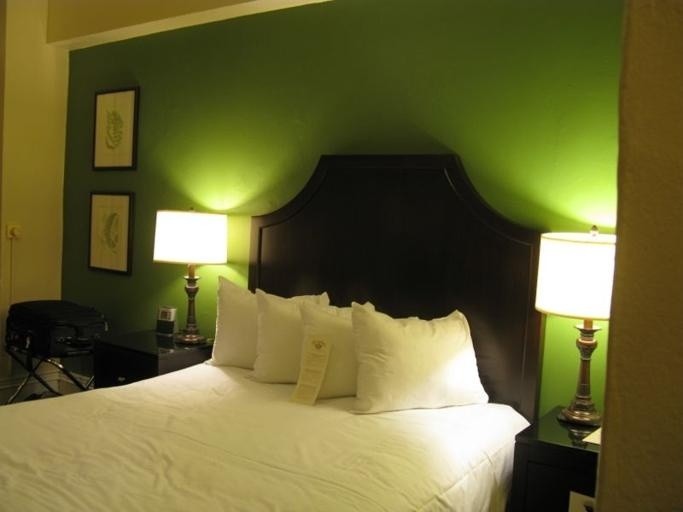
[248, 286, 333, 385]
[292, 300, 377, 401]
[205, 273, 259, 371]
[346, 297, 490, 418]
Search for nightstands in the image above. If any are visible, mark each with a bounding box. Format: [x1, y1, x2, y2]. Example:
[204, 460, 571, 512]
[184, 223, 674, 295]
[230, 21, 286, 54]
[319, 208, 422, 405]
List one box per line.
[88, 327, 215, 392]
[506, 402, 605, 509]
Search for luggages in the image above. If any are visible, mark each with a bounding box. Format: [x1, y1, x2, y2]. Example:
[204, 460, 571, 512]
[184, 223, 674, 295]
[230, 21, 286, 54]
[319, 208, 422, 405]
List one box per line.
[5, 299, 107, 354]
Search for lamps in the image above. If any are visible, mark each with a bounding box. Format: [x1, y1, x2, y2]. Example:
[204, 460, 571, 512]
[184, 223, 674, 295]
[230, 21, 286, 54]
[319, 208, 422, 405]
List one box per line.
[148, 205, 231, 346]
[533, 220, 618, 429]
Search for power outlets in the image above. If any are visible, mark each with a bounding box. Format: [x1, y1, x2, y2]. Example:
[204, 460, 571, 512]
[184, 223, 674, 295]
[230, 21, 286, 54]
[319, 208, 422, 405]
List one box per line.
[5, 223, 22, 239]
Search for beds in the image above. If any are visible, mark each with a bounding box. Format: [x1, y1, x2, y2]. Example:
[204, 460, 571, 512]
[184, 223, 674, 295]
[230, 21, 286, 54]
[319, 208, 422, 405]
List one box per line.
[0, 151, 549, 512]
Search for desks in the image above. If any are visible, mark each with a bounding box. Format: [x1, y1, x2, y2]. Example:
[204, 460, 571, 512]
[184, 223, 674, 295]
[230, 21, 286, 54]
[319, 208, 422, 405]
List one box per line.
[3, 297, 110, 405]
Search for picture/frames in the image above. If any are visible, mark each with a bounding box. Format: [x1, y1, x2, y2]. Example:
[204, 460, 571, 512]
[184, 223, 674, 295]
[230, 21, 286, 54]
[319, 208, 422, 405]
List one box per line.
[90, 84, 138, 171]
[86, 187, 135, 278]
